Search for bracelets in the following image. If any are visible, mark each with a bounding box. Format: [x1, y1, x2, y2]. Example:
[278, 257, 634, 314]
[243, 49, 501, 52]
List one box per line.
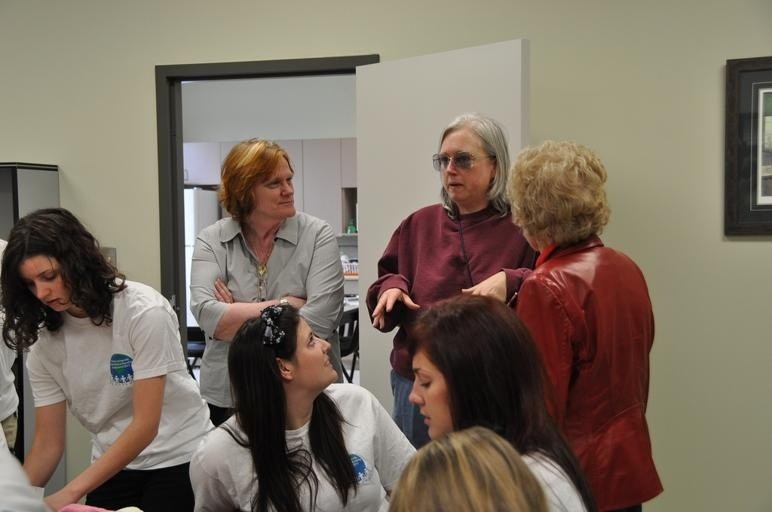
[279, 297, 289, 305]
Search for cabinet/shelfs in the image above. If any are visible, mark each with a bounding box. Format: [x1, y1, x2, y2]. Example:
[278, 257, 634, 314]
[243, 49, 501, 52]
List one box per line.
[183, 142, 222, 186]
[221, 138, 358, 238]
[0, 162, 67, 497]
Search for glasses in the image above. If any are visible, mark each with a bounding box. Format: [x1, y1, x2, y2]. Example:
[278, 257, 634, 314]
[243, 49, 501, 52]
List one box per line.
[432, 151, 493, 172]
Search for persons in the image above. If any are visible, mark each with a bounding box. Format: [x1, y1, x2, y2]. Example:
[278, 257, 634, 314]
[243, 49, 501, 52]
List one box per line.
[390, 427, 550, 512]
[0, 209, 214, 512]
[0, 239, 21, 452]
[513, 137, 664, 511]
[366, 110, 543, 450]
[0, 425, 77, 512]
[409, 292, 596, 512]
[191, 300, 416, 512]
[183, 137, 347, 427]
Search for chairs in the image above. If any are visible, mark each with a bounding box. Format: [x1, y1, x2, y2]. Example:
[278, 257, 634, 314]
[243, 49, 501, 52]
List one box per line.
[338, 308, 359, 383]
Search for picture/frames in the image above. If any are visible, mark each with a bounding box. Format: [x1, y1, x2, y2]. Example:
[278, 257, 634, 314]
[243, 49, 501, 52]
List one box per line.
[726, 56, 772, 235]
